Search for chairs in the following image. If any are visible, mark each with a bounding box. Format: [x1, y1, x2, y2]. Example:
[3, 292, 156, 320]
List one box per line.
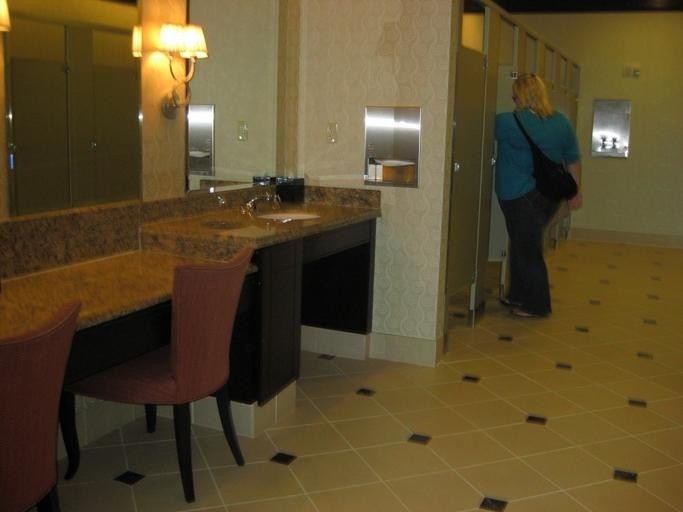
[0, 302, 83, 512]
[59, 242, 254, 503]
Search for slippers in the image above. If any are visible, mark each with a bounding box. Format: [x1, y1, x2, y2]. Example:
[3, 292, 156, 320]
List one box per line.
[510, 306, 547, 319]
[498, 293, 525, 306]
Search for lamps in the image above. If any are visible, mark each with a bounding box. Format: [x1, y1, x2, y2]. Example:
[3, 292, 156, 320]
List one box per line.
[1, 0, 11, 30]
[158, 25, 208, 119]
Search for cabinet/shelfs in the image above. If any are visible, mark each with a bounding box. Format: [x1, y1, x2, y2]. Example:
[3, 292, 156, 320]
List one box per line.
[255, 240, 302, 406]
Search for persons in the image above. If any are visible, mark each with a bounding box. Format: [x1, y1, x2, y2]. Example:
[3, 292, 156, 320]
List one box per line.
[492, 74, 583, 320]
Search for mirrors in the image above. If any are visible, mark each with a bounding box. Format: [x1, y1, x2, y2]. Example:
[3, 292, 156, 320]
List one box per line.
[188, 105, 214, 179]
[363, 105, 421, 188]
[0, 0, 287, 219]
[590, 99, 631, 158]
